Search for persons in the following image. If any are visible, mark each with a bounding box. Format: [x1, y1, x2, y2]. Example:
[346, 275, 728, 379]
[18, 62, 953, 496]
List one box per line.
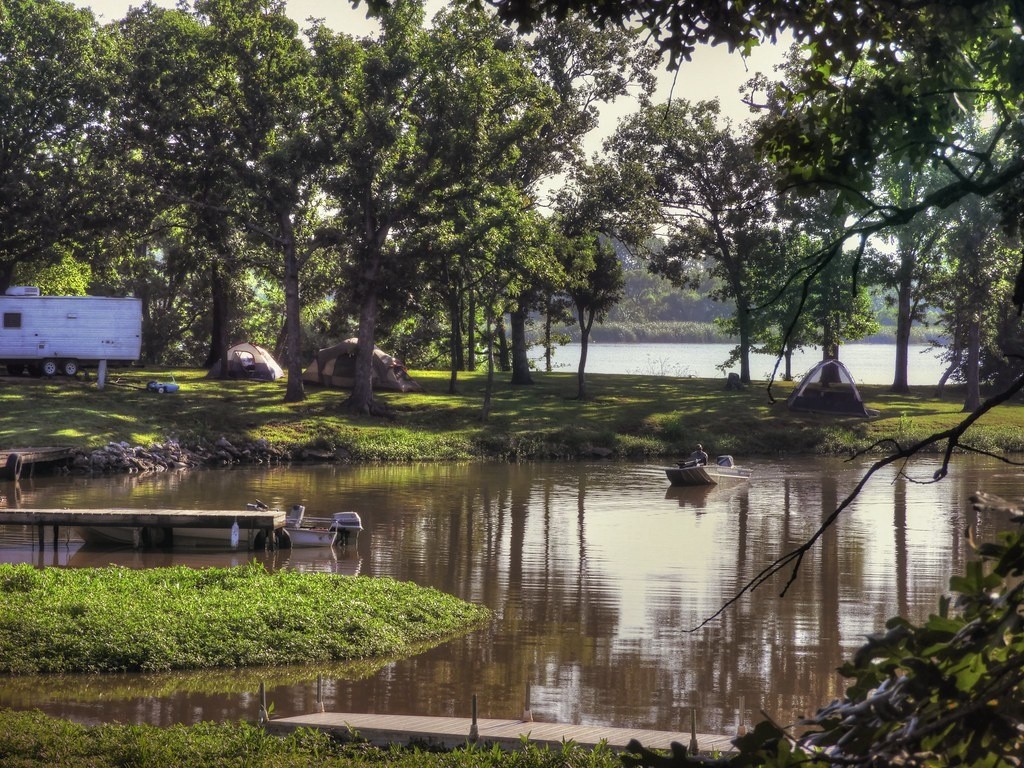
[691, 444, 708, 467]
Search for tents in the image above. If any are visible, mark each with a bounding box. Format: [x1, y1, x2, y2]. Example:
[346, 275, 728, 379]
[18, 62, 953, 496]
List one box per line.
[206, 342, 285, 381]
[788, 358, 870, 417]
[301, 337, 424, 393]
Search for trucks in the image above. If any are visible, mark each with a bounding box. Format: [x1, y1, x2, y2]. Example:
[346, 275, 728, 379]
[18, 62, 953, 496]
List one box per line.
[0, 286, 143, 373]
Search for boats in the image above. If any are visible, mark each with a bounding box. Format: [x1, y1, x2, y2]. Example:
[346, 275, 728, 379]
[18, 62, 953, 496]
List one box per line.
[664, 465, 719, 486]
[79, 499, 363, 548]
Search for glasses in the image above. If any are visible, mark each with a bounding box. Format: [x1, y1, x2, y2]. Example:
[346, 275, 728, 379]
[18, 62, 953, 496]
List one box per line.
[697, 448, 701, 450]
